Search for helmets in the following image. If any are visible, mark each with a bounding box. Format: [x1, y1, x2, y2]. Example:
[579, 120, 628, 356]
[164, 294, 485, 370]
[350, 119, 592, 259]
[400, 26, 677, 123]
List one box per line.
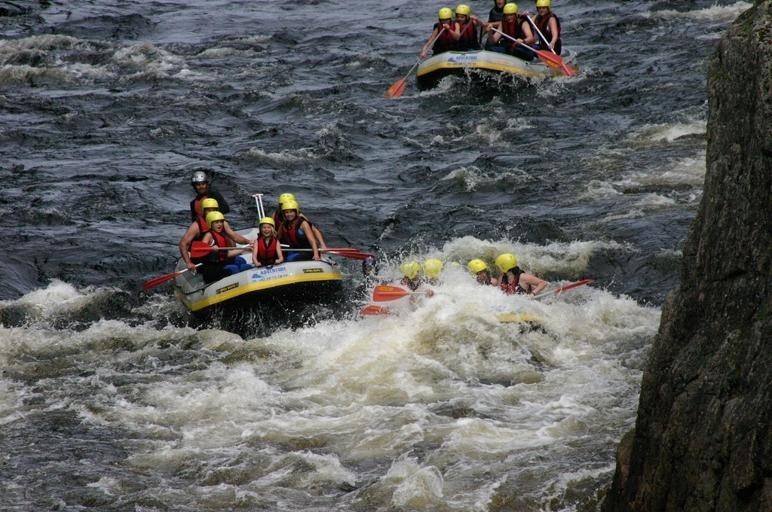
[201, 199, 219, 214]
[206, 211, 224, 229]
[439, 8, 452, 21]
[279, 193, 299, 217]
[495, 253, 517, 273]
[455, 5, 470, 20]
[536, 0, 551, 11]
[192, 172, 207, 185]
[468, 259, 487, 274]
[503, 3, 519, 17]
[259, 217, 275, 229]
[400, 261, 420, 279]
[423, 260, 441, 278]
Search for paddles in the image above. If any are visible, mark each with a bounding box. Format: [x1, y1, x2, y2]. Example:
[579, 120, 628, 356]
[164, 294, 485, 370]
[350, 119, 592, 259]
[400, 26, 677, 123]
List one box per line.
[324, 251, 374, 260]
[190, 242, 252, 258]
[359, 304, 398, 316]
[281, 248, 360, 252]
[145, 263, 204, 289]
[527, 11, 575, 77]
[373, 286, 427, 301]
[529, 278, 593, 298]
[387, 27, 445, 95]
[488, 24, 563, 70]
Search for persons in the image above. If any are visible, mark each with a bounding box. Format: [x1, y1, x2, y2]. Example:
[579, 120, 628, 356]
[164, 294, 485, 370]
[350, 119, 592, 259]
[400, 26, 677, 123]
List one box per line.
[400, 253, 547, 299]
[486, 0, 511, 51]
[521, 0, 562, 59]
[455, 4, 485, 50]
[180, 171, 327, 283]
[485, 2, 536, 61]
[419, 7, 464, 60]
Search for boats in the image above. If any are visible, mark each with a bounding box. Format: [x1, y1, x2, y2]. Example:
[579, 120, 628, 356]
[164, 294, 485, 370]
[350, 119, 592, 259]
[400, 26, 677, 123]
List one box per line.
[413, 38, 589, 102]
[353, 290, 571, 367]
[165, 221, 346, 324]
